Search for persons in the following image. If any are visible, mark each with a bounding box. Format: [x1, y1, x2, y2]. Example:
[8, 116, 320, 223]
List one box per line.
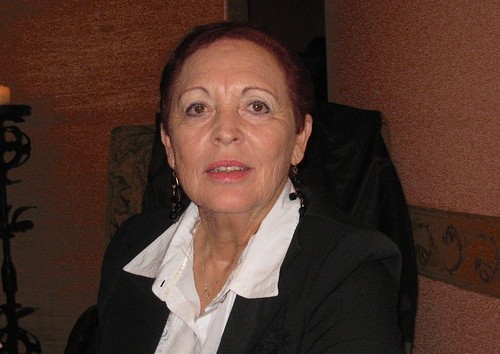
[96, 20, 402, 354]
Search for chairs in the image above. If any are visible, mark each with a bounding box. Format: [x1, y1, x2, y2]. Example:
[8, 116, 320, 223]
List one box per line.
[104, 102, 419, 353]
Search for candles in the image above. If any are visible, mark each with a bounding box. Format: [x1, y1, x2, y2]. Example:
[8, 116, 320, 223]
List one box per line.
[0, 84, 11, 105]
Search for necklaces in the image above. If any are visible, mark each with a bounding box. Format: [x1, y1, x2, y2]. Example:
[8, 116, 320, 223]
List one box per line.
[201, 236, 210, 307]
